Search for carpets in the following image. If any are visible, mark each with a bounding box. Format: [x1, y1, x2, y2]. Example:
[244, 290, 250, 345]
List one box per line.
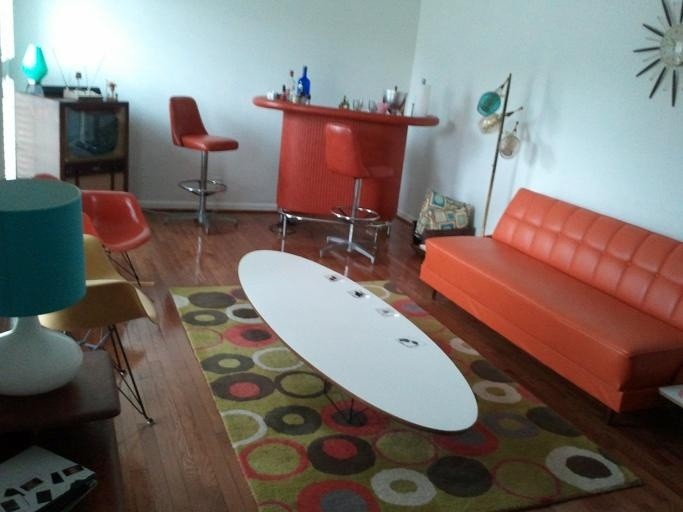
[168, 280, 647, 511]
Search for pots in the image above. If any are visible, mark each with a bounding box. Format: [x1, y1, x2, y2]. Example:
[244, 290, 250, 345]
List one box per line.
[382, 86, 407, 115]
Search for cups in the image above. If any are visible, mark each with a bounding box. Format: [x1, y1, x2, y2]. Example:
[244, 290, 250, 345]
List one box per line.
[367, 95, 380, 113]
[352, 98, 364, 112]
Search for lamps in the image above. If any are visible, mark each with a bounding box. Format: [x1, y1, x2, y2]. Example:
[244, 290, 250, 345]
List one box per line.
[0, 177, 88, 399]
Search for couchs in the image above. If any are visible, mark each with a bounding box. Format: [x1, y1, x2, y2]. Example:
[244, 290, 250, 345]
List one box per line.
[418, 185, 683, 425]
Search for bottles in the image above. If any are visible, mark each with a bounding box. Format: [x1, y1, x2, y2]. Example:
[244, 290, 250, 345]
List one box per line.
[338, 96, 350, 109]
[277, 66, 311, 106]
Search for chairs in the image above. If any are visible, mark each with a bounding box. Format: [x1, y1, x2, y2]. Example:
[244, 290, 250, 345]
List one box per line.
[31, 172, 159, 425]
[319, 122, 395, 265]
[163, 95, 240, 235]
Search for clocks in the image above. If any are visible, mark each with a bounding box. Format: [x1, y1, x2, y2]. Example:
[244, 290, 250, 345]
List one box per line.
[632, 0, 682, 107]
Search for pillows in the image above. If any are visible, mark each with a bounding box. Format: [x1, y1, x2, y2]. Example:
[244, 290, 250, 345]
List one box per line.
[413, 190, 475, 241]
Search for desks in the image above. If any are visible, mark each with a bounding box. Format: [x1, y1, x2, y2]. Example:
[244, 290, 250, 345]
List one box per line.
[1, 350, 127, 511]
[252, 94, 440, 245]
